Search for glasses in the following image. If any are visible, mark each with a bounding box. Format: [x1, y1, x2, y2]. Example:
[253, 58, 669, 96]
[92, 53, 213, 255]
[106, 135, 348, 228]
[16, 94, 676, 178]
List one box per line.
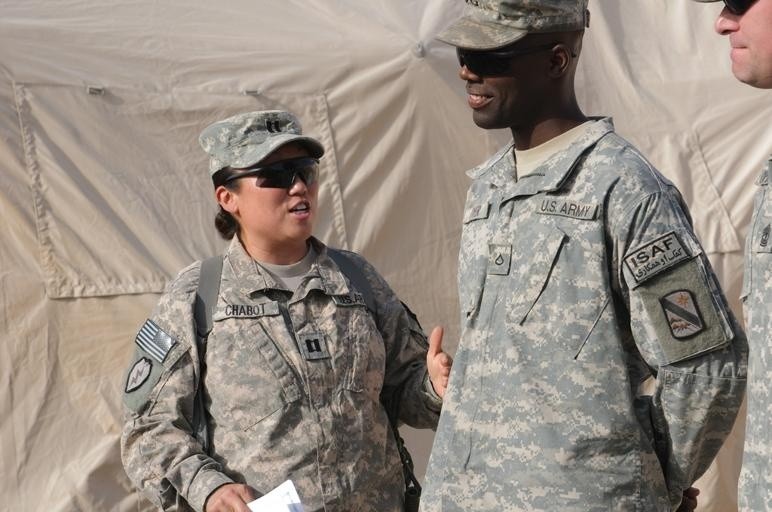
[225, 155, 321, 188]
[456, 40, 577, 78]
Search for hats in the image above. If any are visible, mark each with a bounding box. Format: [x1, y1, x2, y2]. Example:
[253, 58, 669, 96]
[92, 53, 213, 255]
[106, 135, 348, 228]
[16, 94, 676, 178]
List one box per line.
[199, 110, 325, 176]
[434, 0, 591, 51]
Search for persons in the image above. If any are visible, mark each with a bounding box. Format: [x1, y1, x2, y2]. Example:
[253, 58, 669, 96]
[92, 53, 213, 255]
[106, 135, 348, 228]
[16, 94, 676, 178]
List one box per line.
[114, 109, 455, 511]
[712, 0, 772, 512]
[413, 1, 750, 511]
